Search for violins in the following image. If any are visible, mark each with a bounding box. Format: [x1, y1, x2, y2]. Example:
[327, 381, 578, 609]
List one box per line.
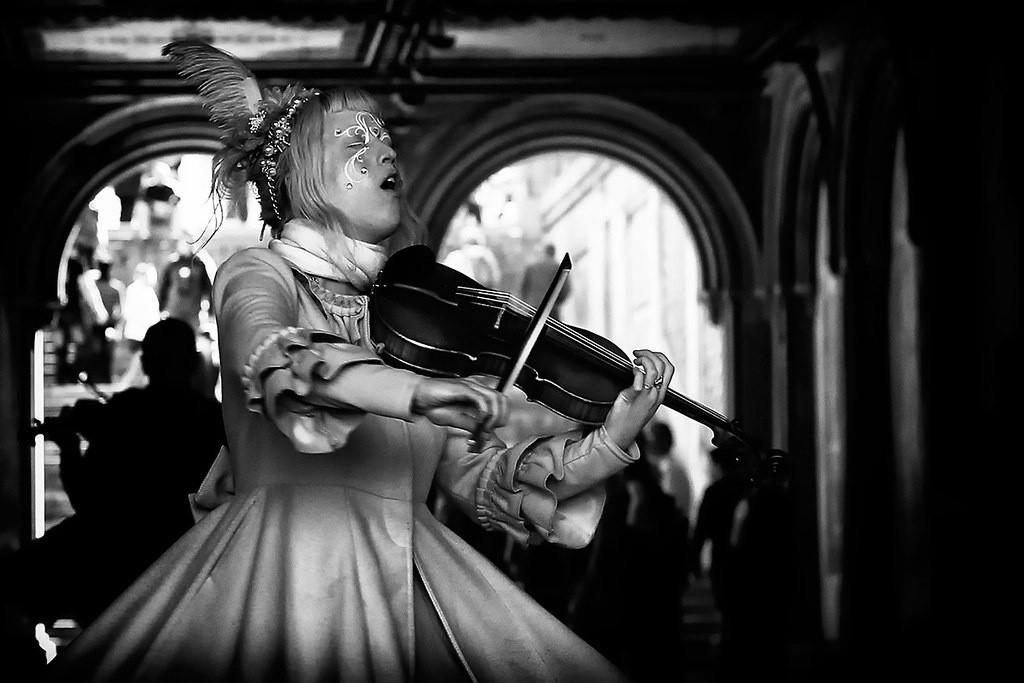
[366, 263, 798, 505]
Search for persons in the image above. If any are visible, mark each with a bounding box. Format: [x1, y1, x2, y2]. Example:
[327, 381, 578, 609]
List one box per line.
[0, 170, 229, 683]
[512, 420, 790, 683]
[43, 39, 676, 683]
[441, 194, 573, 326]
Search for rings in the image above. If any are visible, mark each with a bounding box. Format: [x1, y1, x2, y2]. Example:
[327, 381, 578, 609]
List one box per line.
[654, 376, 665, 389]
[641, 382, 653, 391]
